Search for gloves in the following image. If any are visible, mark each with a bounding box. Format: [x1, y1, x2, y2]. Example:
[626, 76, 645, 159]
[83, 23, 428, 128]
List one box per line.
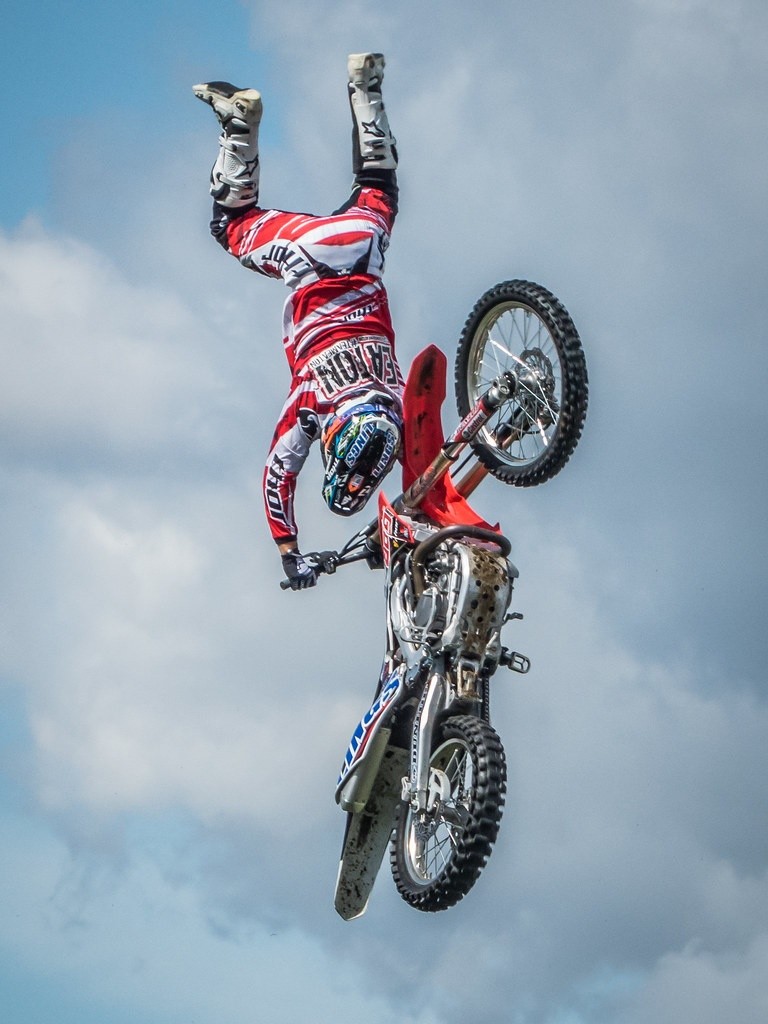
[281, 548, 317, 591]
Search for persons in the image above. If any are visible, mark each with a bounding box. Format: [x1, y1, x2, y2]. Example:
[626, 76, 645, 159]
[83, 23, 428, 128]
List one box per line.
[192, 52, 409, 592]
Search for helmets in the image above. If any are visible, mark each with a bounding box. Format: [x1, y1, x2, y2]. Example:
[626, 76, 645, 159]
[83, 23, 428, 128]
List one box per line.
[320, 389, 402, 516]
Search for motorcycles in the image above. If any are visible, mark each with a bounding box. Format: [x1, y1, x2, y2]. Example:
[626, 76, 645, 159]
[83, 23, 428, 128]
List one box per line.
[281, 276, 590, 922]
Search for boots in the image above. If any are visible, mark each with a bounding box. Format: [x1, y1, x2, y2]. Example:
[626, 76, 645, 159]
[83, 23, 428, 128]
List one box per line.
[193, 81, 263, 209]
[348, 54, 399, 173]
